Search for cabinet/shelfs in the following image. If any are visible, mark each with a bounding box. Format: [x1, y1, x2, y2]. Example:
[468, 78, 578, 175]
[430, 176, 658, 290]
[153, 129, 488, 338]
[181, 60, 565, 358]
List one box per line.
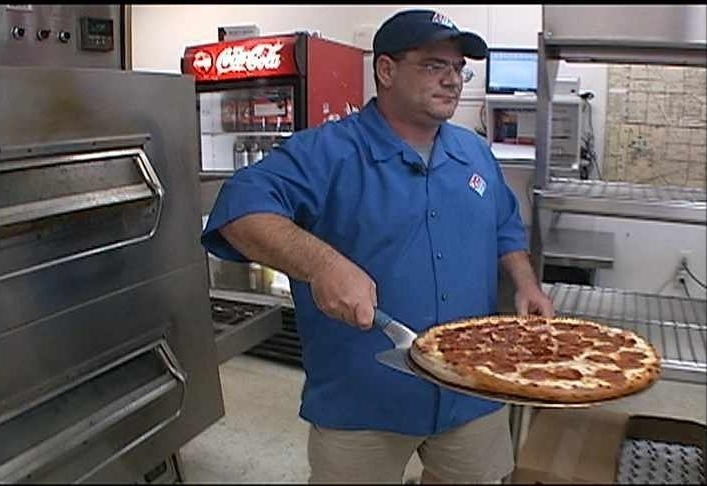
[528, 4, 707, 384]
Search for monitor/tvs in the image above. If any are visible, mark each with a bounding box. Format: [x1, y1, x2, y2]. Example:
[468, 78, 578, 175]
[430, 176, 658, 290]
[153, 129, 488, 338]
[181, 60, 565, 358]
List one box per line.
[485, 48, 540, 94]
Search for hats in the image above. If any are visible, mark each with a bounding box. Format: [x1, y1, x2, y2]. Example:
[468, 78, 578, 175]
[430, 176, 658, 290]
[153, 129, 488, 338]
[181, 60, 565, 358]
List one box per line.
[373, 9, 489, 61]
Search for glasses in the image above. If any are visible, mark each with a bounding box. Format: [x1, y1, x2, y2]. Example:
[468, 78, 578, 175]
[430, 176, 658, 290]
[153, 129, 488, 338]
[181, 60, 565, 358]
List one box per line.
[402, 59, 475, 83]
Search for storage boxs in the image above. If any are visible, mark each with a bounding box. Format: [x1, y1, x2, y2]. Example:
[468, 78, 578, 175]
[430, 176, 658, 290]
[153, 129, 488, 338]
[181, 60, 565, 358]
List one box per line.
[515, 407, 630, 486]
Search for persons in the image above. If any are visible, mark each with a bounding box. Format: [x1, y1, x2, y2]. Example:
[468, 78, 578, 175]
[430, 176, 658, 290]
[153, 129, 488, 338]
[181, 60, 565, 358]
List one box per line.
[201, 9, 554, 485]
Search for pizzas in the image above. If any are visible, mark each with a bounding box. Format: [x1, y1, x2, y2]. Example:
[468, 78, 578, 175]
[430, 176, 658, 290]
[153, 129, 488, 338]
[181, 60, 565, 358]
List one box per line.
[410, 313, 660, 403]
